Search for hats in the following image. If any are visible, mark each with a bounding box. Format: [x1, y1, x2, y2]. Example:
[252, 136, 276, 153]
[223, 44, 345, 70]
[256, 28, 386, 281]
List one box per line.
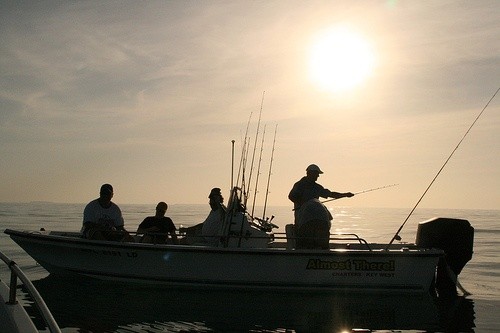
[306, 163, 323, 174]
[208, 187, 221, 198]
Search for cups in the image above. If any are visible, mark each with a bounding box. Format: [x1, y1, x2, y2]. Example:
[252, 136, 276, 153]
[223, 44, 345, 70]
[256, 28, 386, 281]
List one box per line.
[179, 223, 184, 235]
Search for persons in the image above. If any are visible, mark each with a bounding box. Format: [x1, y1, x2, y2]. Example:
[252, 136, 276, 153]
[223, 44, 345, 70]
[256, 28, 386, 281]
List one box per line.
[136, 202, 179, 244]
[288, 163, 353, 250]
[80, 184, 134, 242]
[179, 187, 226, 244]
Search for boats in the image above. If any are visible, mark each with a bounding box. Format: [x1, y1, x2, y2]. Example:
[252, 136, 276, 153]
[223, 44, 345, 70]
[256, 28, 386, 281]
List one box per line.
[3, 217, 475, 296]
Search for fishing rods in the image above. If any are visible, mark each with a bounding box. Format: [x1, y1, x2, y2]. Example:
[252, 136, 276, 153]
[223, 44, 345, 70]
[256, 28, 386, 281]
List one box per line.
[237, 90, 266, 218]
[385, 88, 500, 249]
[291, 183, 399, 211]
[262, 122, 278, 221]
